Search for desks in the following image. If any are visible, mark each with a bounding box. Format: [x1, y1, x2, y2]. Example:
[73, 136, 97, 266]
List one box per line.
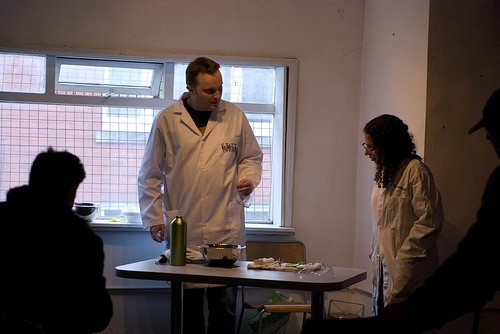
[114, 252, 367, 334]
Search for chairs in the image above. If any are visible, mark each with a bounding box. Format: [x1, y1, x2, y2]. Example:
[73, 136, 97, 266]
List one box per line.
[237, 240, 327, 334]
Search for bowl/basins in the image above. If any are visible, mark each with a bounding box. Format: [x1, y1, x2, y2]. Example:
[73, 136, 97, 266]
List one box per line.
[197, 241, 247, 267]
[123, 211, 141, 223]
[71, 203, 101, 223]
[103, 208, 122, 218]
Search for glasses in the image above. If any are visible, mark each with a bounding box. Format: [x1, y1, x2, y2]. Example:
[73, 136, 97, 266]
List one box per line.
[362, 142, 376, 152]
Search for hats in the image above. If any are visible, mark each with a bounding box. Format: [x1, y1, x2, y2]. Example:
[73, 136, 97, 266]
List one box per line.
[467, 88, 500, 133]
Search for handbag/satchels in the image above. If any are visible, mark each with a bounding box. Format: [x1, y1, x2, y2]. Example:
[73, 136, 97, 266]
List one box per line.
[247, 290, 301, 334]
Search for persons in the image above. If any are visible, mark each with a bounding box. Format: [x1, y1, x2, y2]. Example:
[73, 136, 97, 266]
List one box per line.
[382, 88, 500, 334]
[362, 114, 444, 334]
[0, 146, 114, 334]
[138, 57, 263, 334]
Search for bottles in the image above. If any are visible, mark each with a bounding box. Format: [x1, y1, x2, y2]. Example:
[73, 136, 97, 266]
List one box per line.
[169, 214, 187, 266]
